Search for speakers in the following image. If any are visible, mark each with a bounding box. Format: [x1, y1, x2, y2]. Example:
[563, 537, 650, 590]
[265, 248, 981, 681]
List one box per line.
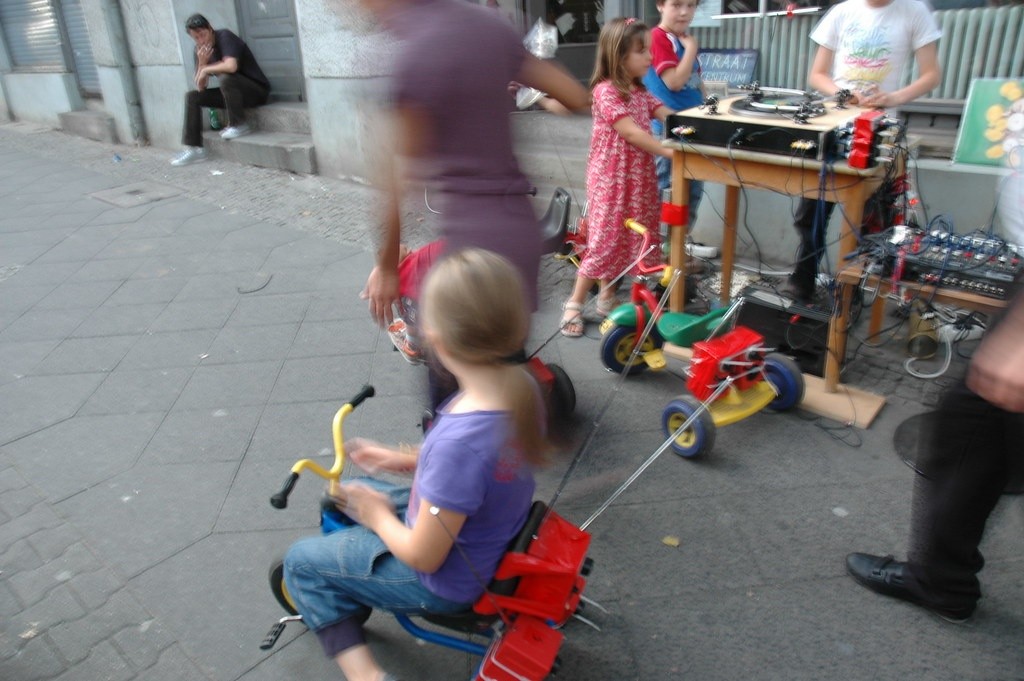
[735, 286, 832, 377]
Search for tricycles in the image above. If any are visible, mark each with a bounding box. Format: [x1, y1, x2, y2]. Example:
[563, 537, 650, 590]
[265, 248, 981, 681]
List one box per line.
[258, 381, 595, 680]
[596, 217, 806, 461]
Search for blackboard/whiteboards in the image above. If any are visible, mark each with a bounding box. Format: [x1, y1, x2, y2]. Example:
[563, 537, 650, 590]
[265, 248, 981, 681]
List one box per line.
[696, 48, 761, 87]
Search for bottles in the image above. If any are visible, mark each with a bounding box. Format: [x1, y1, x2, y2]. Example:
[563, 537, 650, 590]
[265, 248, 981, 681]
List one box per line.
[207, 107, 222, 131]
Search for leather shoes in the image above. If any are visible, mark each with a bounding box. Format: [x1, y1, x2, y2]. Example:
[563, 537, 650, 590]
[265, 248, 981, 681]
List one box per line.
[846, 553, 974, 622]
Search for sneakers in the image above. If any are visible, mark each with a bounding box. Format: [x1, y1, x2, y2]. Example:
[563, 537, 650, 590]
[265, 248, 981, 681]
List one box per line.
[172, 148, 205, 167]
[221, 122, 252, 139]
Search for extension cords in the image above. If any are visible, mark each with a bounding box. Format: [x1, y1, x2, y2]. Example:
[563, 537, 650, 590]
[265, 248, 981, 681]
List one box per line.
[939, 323, 983, 343]
[683, 244, 718, 258]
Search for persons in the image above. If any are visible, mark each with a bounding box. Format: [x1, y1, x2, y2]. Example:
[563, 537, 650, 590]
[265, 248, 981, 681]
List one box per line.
[283, 244, 550, 681]
[636, 1, 705, 255]
[847, 58, 1023, 623]
[168, 13, 270, 167]
[786, 0, 945, 308]
[557, 18, 676, 337]
[355, 0, 590, 427]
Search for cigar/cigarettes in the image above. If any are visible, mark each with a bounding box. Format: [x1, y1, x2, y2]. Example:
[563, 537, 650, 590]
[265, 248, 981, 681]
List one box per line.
[204, 47, 209, 52]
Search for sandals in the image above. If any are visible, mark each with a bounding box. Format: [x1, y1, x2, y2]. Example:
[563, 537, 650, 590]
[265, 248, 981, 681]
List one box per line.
[560, 302, 583, 337]
[596, 297, 617, 316]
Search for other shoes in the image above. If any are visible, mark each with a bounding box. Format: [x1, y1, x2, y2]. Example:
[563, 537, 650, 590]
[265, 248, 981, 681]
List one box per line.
[388, 318, 422, 365]
[778, 275, 809, 297]
[684, 260, 704, 273]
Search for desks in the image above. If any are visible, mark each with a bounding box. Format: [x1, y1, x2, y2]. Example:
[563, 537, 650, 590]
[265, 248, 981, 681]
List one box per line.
[841, 260, 1010, 343]
[664, 132, 922, 393]
